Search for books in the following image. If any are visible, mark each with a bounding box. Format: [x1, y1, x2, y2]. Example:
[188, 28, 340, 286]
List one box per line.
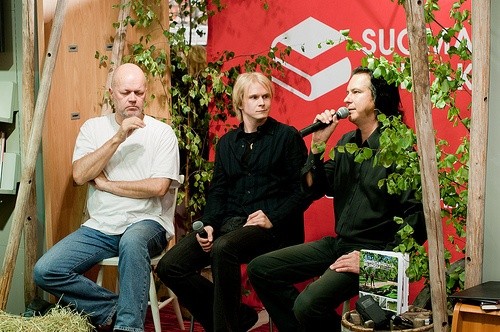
[481, 304, 500, 310]
[359, 249, 410, 317]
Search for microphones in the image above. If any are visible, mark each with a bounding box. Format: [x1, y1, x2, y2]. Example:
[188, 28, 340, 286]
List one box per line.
[299, 107, 349, 138]
[193, 221, 214, 247]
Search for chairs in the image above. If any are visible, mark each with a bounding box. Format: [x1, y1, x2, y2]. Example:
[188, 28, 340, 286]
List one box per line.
[96, 174, 185, 332]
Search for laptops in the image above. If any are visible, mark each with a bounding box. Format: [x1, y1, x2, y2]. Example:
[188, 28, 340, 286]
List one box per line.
[449, 280, 500, 304]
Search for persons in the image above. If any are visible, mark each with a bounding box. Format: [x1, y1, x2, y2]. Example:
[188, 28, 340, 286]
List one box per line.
[156, 72, 309, 332]
[246, 66, 429, 332]
[364, 272, 375, 286]
[32, 63, 185, 332]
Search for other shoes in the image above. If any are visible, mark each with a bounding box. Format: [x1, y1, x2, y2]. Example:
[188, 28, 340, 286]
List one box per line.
[240, 303, 258, 332]
